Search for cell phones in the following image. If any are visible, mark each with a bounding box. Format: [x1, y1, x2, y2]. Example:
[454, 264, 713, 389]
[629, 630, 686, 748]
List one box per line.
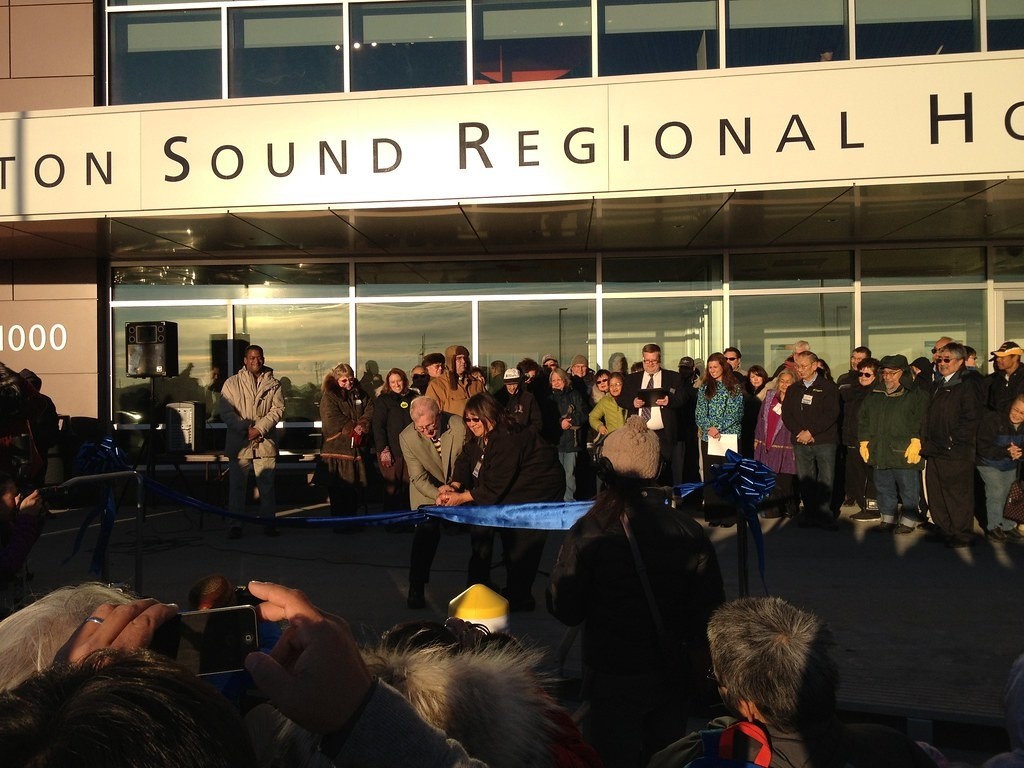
[146, 604, 258, 677]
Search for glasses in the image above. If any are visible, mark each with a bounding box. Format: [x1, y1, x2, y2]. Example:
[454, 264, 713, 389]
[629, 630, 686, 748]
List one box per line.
[597, 379, 607, 384]
[725, 357, 738, 361]
[644, 357, 660, 364]
[707, 667, 723, 686]
[414, 417, 438, 432]
[465, 417, 482, 422]
[338, 378, 354, 383]
[797, 362, 814, 369]
[543, 364, 555, 367]
[857, 348, 977, 378]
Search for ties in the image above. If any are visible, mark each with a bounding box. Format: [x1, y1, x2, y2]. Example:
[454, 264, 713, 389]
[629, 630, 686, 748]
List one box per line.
[641, 373, 654, 423]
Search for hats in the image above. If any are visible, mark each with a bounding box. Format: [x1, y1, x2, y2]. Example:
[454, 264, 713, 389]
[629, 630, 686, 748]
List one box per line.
[991, 342, 1022, 356]
[603, 415, 660, 478]
[422, 345, 587, 390]
[678, 357, 694, 369]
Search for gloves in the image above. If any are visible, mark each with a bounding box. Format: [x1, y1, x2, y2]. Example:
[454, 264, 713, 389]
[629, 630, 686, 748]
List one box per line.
[859, 441, 869, 463]
[350, 425, 362, 446]
[380, 446, 394, 468]
[904, 438, 921, 464]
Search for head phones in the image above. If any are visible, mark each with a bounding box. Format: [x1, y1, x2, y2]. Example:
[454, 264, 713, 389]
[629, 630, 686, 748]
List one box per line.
[592, 429, 664, 483]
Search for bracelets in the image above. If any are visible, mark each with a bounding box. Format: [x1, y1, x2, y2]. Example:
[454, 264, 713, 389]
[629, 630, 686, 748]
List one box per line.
[450, 486, 457, 491]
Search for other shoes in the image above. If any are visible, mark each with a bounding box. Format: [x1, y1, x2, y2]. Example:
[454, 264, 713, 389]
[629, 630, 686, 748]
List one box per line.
[263, 526, 280, 537]
[228, 528, 241, 540]
[408, 585, 426, 608]
[708, 503, 1024, 549]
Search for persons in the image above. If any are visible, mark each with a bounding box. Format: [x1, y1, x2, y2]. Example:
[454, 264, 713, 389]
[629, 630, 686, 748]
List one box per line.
[647, 596, 939, 768]
[0, 579, 490, 768]
[0, 361, 59, 519]
[399, 396, 500, 609]
[438, 392, 566, 613]
[372, 368, 420, 534]
[0, 477, 43, 582]
[545, 415, 728, 768]
[316, 364, 375, 535]
[980, 342, 1024, 411]
[409, 340, 930, 534]
[220, 345, 286, 538]
[963, 346, 978, 370]
[248, 615, 604, 768]
[913, 337, 956, 532]
[975, 393, 1024, 544]
[919, 342, 983, 548]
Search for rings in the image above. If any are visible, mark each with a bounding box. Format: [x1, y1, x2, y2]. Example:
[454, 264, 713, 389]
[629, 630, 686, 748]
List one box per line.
[85, 617, 104, 624]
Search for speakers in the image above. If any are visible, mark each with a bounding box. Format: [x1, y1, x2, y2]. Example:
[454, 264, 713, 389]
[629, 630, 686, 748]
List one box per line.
[166, 400, 206, 453]
[125, 320, 179, 380]
